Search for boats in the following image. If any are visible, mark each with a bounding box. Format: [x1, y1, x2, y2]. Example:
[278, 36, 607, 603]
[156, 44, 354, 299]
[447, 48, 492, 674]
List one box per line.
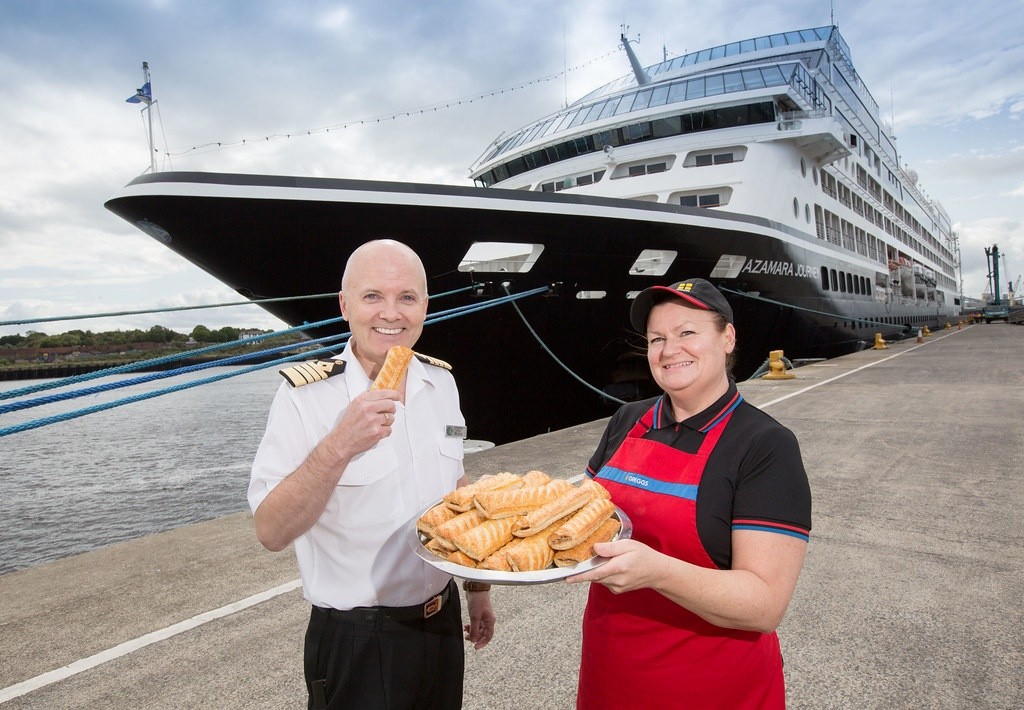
[103, 0, 982, 446]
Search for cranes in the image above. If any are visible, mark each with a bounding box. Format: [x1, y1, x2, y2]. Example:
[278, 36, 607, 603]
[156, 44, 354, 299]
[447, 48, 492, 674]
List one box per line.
[983, 243, 1024, 325]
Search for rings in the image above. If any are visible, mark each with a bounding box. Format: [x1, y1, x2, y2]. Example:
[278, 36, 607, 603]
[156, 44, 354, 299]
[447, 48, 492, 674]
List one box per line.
[383, 413, 389, 426]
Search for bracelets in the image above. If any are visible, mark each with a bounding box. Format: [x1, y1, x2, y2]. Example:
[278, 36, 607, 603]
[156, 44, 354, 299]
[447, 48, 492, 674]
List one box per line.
[462, 580, 491, 592]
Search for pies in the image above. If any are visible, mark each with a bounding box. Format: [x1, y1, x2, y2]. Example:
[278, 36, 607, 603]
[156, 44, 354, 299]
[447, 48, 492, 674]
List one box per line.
[417, 469, 624, 572]
[371, 344, 414, 391]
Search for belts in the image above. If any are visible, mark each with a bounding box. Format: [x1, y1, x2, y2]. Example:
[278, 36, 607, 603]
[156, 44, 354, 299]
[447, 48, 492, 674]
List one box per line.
[312, 576, 455, 624]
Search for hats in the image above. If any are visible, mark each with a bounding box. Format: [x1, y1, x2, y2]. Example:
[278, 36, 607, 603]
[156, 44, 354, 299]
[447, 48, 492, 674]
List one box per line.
[630, 278, 733, 337]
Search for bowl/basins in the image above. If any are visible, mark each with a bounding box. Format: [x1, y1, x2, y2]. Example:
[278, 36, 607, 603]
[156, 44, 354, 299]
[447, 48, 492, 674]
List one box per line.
[406, 498, 633, 586]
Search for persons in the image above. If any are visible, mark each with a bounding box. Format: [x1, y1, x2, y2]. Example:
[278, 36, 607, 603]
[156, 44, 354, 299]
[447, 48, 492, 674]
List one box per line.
[247, 239, 497, 710]
[565, 278, 813, 710]
